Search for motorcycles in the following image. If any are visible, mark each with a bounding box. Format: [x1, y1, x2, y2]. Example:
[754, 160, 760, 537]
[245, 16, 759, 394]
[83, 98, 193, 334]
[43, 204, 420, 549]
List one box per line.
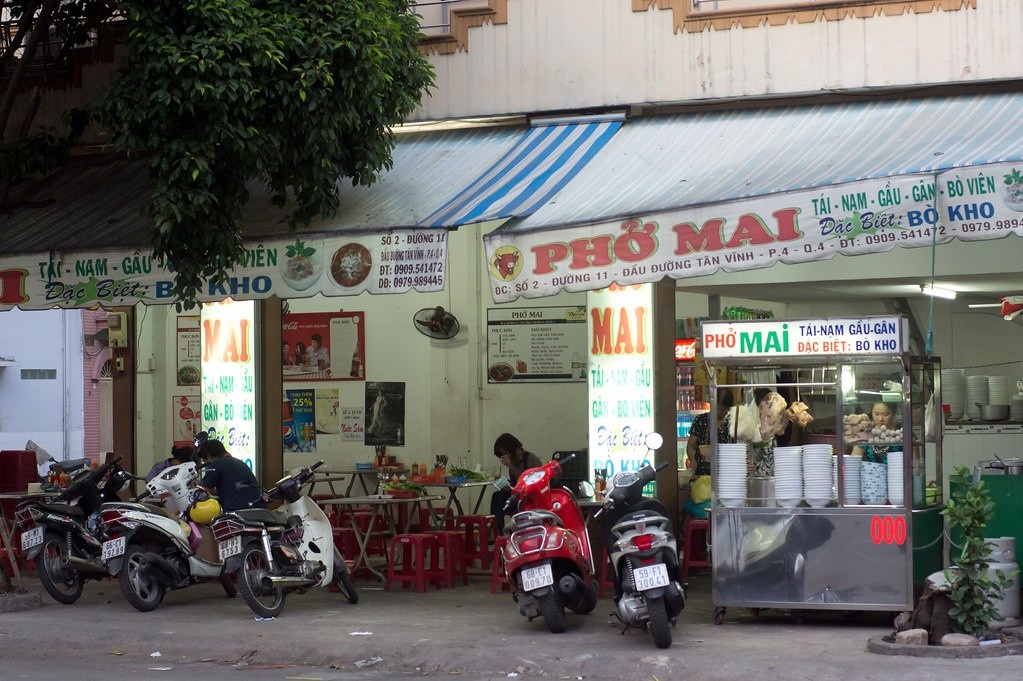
[578, 432, 689, 649]
[100, 459, 246, 613]
[14, 456, 146, 604]
[492, 453, 601, 634]
[209, 459, 359, 618]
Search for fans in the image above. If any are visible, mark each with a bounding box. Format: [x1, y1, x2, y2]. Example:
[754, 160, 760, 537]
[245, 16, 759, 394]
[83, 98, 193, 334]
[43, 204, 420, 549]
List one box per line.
[412, 306, 460, 384]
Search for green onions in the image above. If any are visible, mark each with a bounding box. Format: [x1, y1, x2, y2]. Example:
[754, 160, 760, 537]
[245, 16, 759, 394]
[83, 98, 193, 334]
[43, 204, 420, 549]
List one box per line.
[447, 465, 491, 479]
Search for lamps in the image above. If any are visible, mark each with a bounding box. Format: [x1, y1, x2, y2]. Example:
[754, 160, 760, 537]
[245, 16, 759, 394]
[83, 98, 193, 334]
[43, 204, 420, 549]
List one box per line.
[920, 284, 958, 301]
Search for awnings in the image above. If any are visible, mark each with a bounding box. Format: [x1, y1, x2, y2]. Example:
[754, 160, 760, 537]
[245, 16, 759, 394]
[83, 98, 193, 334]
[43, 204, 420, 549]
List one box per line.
[482, 90, 1022, 304]
[1, 122, 621, 312]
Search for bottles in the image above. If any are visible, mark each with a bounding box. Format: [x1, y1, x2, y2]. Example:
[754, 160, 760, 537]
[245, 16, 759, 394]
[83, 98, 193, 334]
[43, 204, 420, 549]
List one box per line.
[48, 471, 71, 488]
[399, 474, 406, 485]
[723, 306, 774, 321]
[300, 422, 314, 441]
[392, 474, 398, 484]
[420, 464, 427, 477]
[350, 342, 360, 377]
[282, 391, 297, 448]
[412, 460, 418, 475]
[675, 367, 702, 439]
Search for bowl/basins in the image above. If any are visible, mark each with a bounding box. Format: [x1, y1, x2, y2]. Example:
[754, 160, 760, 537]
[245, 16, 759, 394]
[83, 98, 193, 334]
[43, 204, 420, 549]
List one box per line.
[926, 488, 937, 504]
[356, 463, 373, 469]
[941, 368, 1023, 421]
[717, 443, 748, 508]
[446, 476, 465, 484]
[806, 434, 836, 446]
[999, 174, 1023, 212]
[280, 255, 323, 291]
[773, 444, 904, 508]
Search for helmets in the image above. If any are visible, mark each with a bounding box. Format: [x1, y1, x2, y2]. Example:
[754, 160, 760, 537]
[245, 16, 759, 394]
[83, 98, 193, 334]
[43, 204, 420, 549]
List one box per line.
[189, 498, 224, 524]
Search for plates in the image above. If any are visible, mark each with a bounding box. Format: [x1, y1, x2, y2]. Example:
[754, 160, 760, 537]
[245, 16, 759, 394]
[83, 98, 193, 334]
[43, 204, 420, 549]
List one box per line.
[327, 241, 374, 290]
[178, 365, 200, 384]
[489, 362, 514, 382]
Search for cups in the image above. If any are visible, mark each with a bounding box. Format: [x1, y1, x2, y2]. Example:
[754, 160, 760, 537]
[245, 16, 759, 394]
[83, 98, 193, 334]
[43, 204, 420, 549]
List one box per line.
[317, 359, 325, 369]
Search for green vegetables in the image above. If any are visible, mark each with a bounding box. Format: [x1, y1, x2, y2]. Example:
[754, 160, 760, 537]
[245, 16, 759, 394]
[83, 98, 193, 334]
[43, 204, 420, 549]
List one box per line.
[386, 482, 428, 496]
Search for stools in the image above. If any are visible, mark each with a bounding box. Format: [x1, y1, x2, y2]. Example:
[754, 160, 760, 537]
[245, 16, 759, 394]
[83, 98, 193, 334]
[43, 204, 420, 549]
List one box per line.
[680, 515, 708, 578]
[456, 513, 501, 570]
[490, 535, 509, 594]
[598, 544, 614, 599]
[314, 493, 468, 593]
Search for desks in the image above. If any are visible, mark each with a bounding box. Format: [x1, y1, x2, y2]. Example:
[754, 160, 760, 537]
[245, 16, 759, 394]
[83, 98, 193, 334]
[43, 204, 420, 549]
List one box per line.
[316, 495, 446, 590]
[314, 468, 410, 524]
[414, 479, 494, 564]
[576, 496, 604, 526]
[304, 475, 349, 527]
[129, 496, 218, 502]
[0, 491, 61, 590]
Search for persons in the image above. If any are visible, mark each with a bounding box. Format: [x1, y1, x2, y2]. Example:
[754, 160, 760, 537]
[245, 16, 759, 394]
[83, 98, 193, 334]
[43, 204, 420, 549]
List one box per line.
[196, 440, 260, 513]
[491, 433, 542, 535]
[282, 334, 329, 366]
[853, 401, 921, 474]
[145, 445, 194, 483]
[182, 420, 193, 441]
[682, 389, 736, 536]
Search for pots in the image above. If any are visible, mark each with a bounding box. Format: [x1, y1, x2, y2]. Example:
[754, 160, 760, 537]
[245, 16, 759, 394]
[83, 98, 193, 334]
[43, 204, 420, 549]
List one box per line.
[974, 401, 1010, 421]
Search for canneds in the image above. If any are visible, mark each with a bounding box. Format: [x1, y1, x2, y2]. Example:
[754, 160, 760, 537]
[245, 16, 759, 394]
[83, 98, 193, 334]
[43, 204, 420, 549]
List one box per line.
[283, 419, 297, 446]
[694, 385, 702, 401]
